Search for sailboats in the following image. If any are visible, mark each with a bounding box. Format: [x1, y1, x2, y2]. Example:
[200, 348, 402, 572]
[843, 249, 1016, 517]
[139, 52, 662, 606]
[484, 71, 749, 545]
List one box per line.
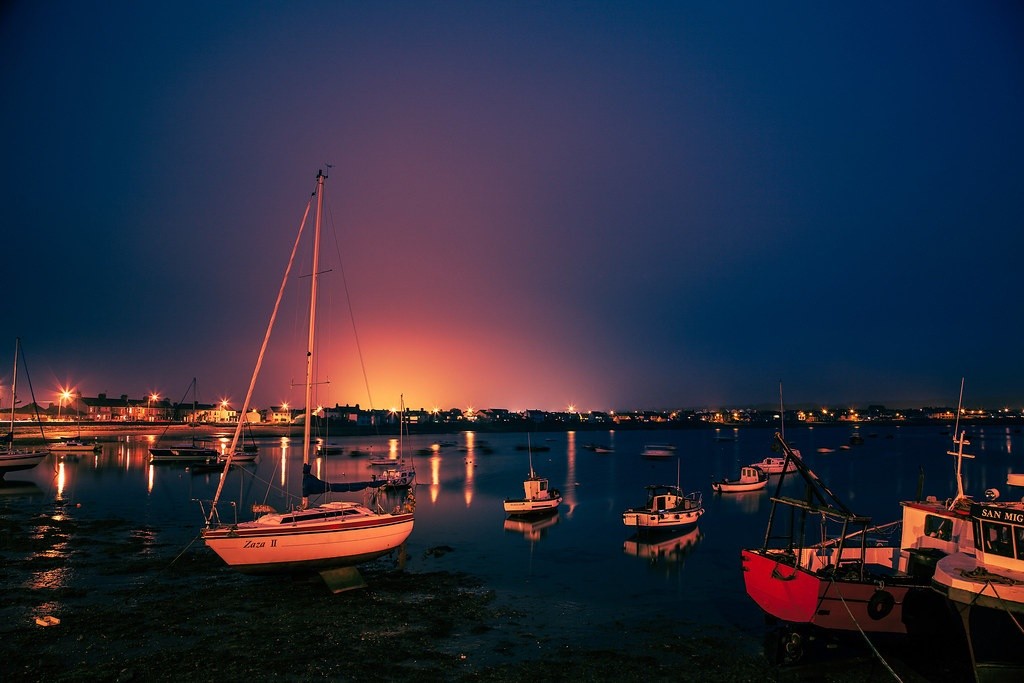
[198, 170, 414, 576]
[0, 335, 52, 478]
[219, 403, 259, 461]
[147, 377, 219, 465]
[371, 393, 416, 488]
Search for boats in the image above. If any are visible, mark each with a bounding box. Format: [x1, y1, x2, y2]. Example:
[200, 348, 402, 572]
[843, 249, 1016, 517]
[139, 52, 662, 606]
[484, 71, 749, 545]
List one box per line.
[620, 456, 705, 528]
[504, 433, 563, 517]
[932, 473, 1024, 683]
[593, 446, 615, 454]
[623, 523, 700, 559]
[417, 447, 435, 456]
[816, 435, 864, 453]
[191, 449, 233, 475]
[740, 375, 986, 652]
[503, 508, 560, 568]
[302, 437, 345, 456]
[640, 445, 679, 456]
[711, 465, 770, 492]
[47, 435, 104, 453]
[749, 446, 802, 474]
